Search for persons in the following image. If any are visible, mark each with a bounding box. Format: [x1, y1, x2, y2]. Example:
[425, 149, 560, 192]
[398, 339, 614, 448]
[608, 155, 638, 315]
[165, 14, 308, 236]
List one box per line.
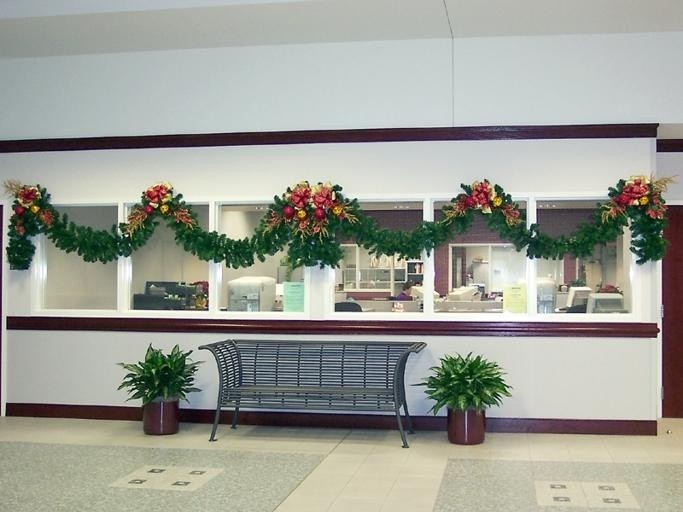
[398, 281, 414, 299]
[415, 281, 420, 287]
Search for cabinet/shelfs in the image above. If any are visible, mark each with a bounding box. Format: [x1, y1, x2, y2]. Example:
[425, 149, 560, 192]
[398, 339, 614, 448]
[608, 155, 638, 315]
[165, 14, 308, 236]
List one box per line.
[336, 244, 425, 294]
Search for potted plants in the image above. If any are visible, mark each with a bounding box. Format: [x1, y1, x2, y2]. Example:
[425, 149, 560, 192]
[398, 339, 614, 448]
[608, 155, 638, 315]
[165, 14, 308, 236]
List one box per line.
[406, 350, 516, 448]
[116, 341, 208, 437]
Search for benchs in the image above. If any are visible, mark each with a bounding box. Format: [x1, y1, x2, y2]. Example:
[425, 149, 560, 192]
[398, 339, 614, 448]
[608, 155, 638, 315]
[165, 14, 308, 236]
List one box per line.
[197, 338, 427, 449]
